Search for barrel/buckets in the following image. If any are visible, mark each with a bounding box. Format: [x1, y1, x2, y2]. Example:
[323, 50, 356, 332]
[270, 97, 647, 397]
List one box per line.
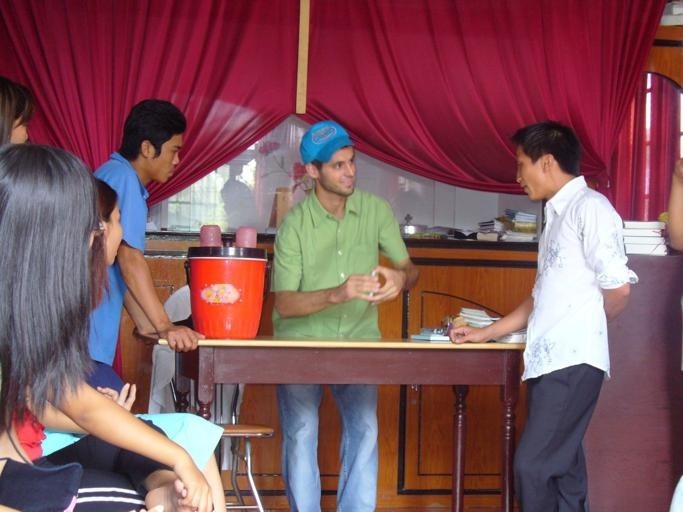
[185, 246, 272, 340]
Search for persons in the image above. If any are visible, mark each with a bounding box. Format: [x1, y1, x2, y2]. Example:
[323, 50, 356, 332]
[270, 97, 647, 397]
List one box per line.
[85, 97, 206, 397]
[0, 72, 39, 158]
[265, 119, 419, 511]
[0, 136, 215, 512]
[10, 175, 230, 511]
[445, 119, 635, 512]
[666, 154, 683, 258]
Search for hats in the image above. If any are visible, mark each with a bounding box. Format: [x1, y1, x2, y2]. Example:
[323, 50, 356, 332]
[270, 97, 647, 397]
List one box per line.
[300, 120, 353, 164]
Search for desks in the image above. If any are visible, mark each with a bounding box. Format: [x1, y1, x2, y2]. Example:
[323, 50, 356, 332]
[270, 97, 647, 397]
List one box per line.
[135, 336, 525, 512]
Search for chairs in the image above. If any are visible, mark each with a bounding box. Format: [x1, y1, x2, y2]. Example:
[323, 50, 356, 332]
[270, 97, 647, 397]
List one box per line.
[168, 373, 276, 512]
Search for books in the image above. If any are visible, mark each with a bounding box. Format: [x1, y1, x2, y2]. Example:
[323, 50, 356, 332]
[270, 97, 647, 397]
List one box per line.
[662, 0, 683, 15]
[398, 206, 537, 241]
[410, 306, 526, 344]
[659, 15, 683, 25]
[622, 217, 668, 258]
